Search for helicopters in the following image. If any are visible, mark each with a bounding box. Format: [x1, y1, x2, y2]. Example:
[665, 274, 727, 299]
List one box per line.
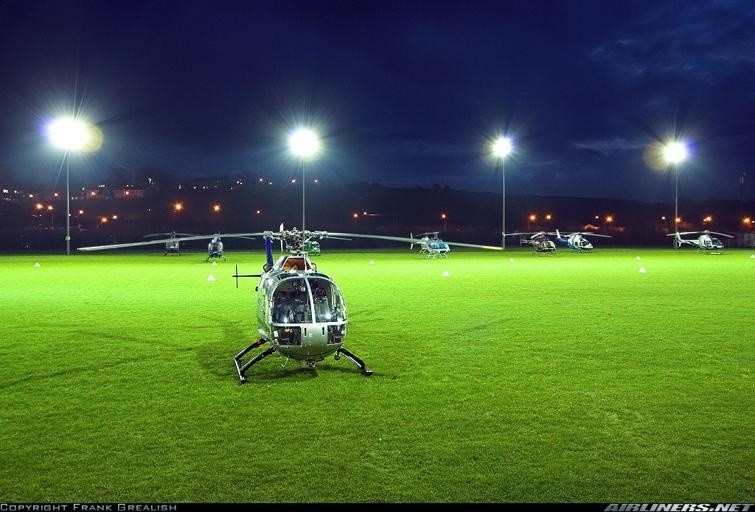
[667, 229, 735, 255]
[502, 229, 613, 252]
[76, 227, 502, 382]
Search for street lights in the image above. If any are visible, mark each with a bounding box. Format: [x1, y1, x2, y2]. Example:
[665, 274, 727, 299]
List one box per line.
[296, 127, 311, 252]
[670, 144, 680, 254]
[736, 170, 746, 250]
[59, 123, 76, 255]
[443, 214, 445, 235]
[496, 140, 508, 253]
